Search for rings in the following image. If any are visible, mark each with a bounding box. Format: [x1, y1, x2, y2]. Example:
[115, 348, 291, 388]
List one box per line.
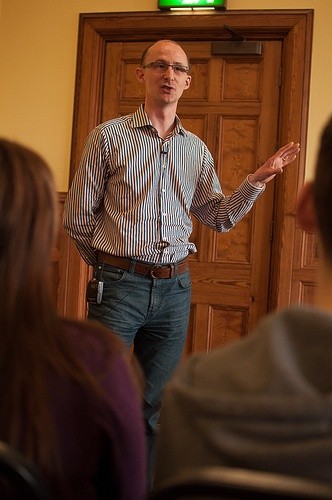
[282, 158, 287, 161]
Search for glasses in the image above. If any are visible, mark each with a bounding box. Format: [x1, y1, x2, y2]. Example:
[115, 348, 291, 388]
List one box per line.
[141, 62, 190, 76]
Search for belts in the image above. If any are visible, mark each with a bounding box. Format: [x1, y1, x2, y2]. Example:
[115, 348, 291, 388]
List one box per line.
[96, 251, 189, 279]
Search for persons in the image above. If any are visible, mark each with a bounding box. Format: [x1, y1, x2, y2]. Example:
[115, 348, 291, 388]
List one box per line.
[0, 138, 149, 500]
[153, 112, 332, 492]
[62, 35, 301, 452]
